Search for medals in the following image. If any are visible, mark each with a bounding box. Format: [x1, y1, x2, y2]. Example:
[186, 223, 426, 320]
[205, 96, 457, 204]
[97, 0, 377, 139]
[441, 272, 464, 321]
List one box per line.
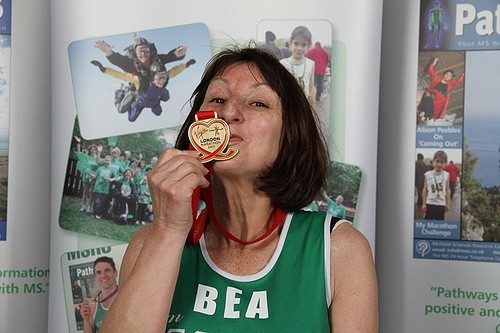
[188, 110, 240, 164]
[99, 45, 380, 333]
[87, 298, 94, 306]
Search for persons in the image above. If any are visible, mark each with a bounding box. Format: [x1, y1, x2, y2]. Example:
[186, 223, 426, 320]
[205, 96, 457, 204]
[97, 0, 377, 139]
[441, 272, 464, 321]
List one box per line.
[89, 35, 197, 123]
[414, 150, 461, 221]
[259, 25, 329, 115]
[73, 256, 119, 333]
[415, 56, 464, 126]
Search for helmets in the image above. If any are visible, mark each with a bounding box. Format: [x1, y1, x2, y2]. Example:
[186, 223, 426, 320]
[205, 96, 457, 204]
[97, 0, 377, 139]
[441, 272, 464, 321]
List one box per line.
[149, 62, 167, 81]
[133, 37, 149, 51]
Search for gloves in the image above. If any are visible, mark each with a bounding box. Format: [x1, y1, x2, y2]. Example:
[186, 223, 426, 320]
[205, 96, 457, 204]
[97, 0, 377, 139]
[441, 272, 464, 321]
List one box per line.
[90, 60, 106, 72]
[94, 39, 115, 56]
[186, 59, 195, 67]
[174, 44, 188, 56]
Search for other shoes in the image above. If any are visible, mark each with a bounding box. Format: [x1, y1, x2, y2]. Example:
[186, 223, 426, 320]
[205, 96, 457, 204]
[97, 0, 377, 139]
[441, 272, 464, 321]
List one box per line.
[87, 209, 90, 212]
[119, 217, 127, 224]
[142, 221, 145, 225]
[79, 208, 84, 211]
[135, 221, 139, 224]
[96, 215, 102, 219]
